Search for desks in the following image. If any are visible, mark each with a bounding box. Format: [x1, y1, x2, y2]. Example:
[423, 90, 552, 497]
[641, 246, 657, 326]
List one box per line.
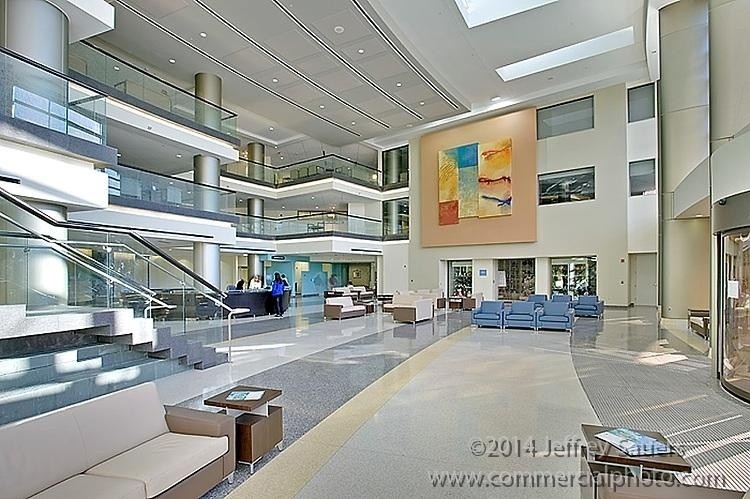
[580, 423, 691, 499]
[595, 472, 750, 499]
[204, 385, 284, 474]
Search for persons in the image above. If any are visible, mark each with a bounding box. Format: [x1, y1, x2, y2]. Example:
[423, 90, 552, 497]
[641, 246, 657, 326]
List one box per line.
[272, 273, 284, 317]
[281, 275, 291, 287]
[236, 279, 244, 290]
[248, 274, 262, 289]
[329, 274, 339, 285]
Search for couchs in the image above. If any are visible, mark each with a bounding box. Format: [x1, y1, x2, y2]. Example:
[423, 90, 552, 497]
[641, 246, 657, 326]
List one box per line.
[0, 382, 237, 499]
[503, 302, 537, 331]
[549, 295, 572, 308]
[526, 295, 547, 304]
[471, 301, 503, 330]
[536, 302, 575, 332]
[572, 295, 604, 319]
[382, 289, 443, 324]
[688, 308, 710, 340]
[323, 285, 377, 320]
